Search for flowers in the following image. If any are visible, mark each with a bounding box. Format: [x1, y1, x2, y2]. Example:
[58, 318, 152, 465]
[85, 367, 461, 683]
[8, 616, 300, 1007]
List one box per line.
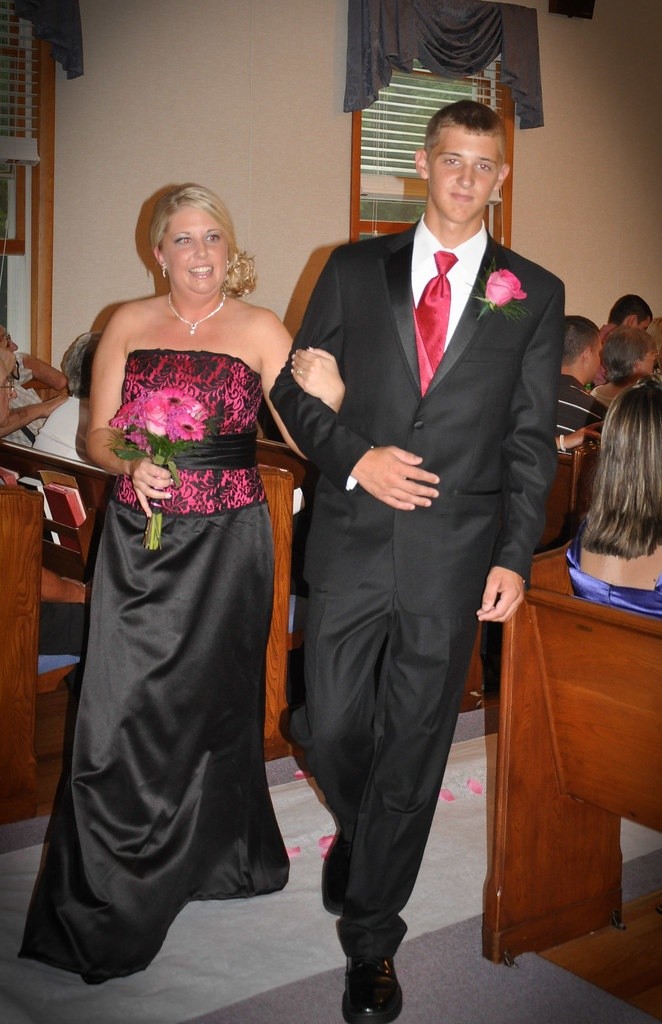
[465, 255, 533, 323]
[102, 385, 224, 550]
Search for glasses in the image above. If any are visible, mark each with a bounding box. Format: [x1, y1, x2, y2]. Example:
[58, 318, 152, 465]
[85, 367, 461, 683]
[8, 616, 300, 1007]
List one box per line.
[0, 379, 14, 392]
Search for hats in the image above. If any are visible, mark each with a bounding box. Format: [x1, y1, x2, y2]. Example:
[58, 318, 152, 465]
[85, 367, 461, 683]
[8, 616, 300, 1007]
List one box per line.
[0, 348, 16, 386]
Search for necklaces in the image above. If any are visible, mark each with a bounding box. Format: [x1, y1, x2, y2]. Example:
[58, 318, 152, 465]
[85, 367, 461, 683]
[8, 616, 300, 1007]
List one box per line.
[169, 292, 225, 335]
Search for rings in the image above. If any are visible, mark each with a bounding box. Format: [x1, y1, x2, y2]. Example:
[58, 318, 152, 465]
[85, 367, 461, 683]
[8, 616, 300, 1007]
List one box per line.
[295, 370, 303, 376]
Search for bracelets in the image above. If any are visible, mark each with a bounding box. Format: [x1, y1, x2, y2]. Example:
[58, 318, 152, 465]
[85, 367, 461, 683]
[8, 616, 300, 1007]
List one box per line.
[560, 434, 566, 451]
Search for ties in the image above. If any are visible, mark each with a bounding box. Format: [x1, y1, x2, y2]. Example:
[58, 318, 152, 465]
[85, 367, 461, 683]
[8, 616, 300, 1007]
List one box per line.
[415, 251, 456, 373]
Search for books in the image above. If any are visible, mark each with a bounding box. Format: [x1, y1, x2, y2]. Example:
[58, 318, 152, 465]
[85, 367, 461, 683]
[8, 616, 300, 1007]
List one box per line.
[0, 467, 86, 553]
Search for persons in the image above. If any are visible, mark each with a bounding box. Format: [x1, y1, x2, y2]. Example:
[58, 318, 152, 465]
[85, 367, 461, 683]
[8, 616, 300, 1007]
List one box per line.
[269, 99, 565, 1020]
[0, 328, 103, 463]
[556, 292, 662, 453]
[17, 182, 348, 987]
[564, 381, 662, 617]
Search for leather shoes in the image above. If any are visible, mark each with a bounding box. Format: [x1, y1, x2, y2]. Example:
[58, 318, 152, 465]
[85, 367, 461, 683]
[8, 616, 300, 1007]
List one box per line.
[321, 827, 354, 915]
[342, 956, 402, 1024]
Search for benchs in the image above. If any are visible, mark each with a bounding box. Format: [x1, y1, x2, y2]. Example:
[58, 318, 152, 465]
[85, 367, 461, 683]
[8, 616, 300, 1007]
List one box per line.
[535, 440, 598, 552]
[0, 483, 81, 826]
[0, 439, 301, 763]
[482, 535, 662, 971]
